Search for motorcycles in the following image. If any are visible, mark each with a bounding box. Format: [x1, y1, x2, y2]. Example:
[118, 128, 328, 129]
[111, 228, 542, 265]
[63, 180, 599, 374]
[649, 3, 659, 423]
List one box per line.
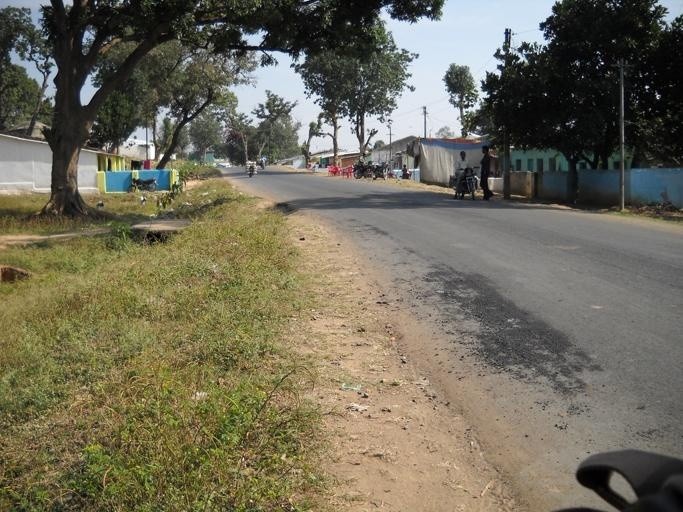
[352, 162, 388, 180]
[127, 177, 156, 194]
[246, 161, 257, 178]
[450, 165, 483, 200]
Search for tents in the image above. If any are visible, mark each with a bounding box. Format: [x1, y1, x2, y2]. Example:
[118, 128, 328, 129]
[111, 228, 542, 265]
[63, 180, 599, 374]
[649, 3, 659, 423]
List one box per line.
[402, 136, 490, 187]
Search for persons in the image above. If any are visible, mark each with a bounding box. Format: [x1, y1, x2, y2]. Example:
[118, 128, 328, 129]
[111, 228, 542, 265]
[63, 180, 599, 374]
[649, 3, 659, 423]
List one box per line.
[259, 154, 266, 165]
[402, 164, 407, 180]
[479, 145, 494, 201]
[453, 150, 474, 200]
